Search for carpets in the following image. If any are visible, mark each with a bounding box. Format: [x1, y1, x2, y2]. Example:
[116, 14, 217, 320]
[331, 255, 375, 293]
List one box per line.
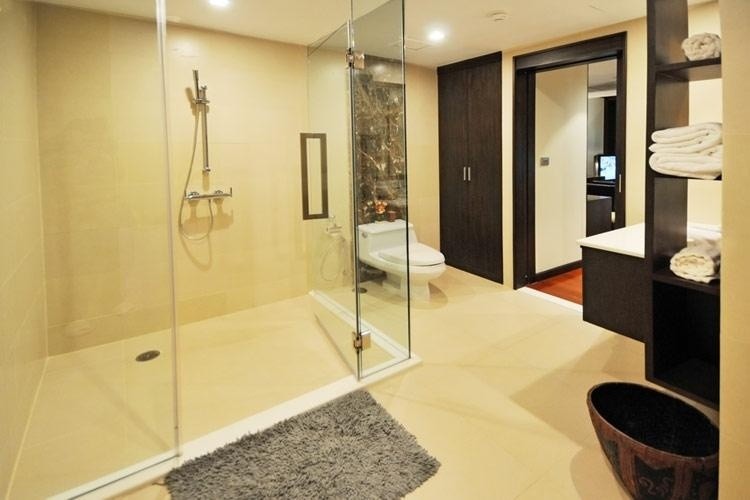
[162, 387, 441, 500]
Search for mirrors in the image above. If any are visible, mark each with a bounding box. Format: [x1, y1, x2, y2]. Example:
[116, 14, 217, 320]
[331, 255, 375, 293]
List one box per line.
[300, 133, 328, 220]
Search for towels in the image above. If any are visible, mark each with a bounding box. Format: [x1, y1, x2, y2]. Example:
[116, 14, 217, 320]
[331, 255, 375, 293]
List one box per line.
[670, 238, 721, 283]
[680, 32, 721, 62]
[647, 122, 722, 180]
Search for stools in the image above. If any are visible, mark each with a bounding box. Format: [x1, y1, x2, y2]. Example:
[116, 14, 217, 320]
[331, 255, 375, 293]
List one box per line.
[585, 381, 719, 500]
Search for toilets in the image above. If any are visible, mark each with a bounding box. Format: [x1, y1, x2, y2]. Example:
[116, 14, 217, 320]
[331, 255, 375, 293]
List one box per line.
[356, 219, 446, 301]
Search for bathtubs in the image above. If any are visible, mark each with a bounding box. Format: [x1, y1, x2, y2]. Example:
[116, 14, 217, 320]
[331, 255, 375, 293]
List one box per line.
[576, 222, 721, 260]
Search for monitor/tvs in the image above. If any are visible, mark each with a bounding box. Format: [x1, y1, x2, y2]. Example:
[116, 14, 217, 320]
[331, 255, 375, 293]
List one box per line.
[595, 154, 617, 180]
